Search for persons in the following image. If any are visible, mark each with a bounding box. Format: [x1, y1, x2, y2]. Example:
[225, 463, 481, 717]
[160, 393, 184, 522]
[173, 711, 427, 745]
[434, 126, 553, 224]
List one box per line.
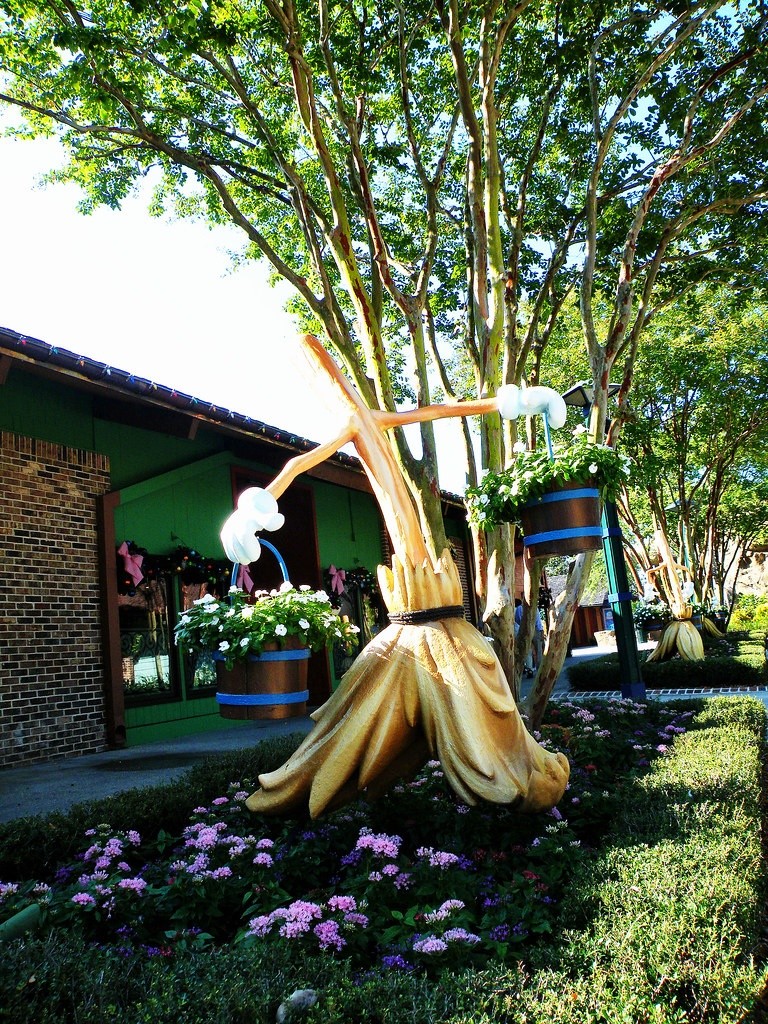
[513, 595, 545, 680]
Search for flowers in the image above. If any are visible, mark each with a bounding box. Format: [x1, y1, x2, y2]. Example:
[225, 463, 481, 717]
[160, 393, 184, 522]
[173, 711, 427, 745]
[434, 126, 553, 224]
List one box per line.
[173, 580, 361, 671]
[461, 420, 634, 530]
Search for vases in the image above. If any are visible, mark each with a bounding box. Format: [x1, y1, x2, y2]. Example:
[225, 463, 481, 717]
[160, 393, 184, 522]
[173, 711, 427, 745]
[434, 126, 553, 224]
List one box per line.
[516, 474, 603, 558]
[213, 634, 313, 719]
